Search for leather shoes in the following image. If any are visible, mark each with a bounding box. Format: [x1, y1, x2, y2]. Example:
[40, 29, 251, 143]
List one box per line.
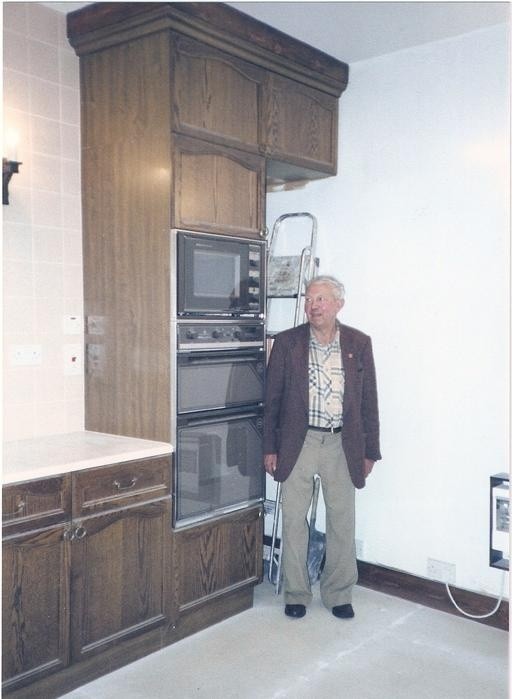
[284, 604, 306, 618]
[332, 604, 354, 618]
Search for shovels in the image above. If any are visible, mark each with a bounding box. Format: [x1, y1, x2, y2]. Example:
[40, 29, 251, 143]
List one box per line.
[306, 478, 326, 585]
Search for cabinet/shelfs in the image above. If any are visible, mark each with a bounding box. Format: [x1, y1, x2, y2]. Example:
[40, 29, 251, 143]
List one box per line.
[62, 4, 351, 644]
[3, 452, 173, 699]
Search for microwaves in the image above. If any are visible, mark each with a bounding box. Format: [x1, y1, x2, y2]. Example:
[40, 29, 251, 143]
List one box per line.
[171, 229, 268, 321]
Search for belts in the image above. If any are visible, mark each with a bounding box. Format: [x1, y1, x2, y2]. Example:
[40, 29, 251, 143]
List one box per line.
[307, 424, 343, 434]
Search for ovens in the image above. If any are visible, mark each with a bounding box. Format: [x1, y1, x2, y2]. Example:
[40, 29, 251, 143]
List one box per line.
[175, 349, 267, 526]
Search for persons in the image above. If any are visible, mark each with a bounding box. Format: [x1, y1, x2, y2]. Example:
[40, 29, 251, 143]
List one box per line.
[223, 278, 262, 500]
[264, 276, 380, 618]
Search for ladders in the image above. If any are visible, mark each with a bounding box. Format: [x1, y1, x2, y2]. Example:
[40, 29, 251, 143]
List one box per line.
[262, 212, 319, 596]
